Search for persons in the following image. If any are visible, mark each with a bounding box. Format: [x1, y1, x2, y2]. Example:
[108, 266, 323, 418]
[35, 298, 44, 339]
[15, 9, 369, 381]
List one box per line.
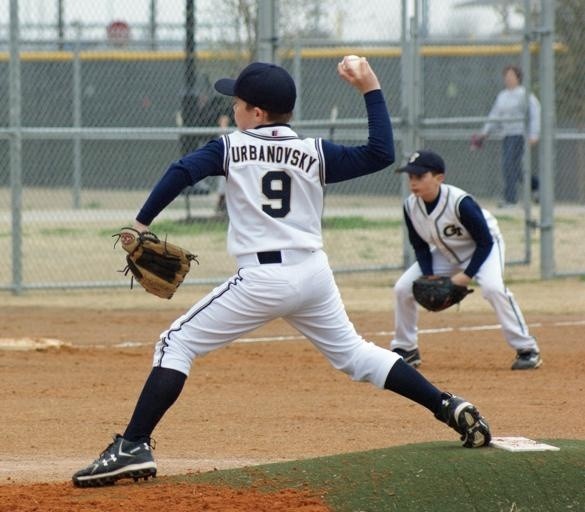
[472, 63, 543, 207]
[172, 74, 236, 195]
[71, 53, 493, 490]
[387, 148, 544, 371]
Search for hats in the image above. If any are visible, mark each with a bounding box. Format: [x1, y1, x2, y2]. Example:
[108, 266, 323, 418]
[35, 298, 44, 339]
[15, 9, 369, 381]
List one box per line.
[394, 151, 445, 175]
[214, 62, 295, 113]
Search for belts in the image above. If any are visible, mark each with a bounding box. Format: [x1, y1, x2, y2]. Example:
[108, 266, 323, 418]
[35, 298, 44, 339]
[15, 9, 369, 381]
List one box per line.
[257, 251, 281, 264]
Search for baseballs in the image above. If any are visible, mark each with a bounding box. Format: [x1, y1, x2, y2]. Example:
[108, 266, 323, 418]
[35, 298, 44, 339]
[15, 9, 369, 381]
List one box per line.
[341, 54, 363, 74]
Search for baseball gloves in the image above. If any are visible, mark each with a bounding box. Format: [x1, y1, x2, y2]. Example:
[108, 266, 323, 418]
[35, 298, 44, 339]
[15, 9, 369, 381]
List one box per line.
[413, 275, 474, 312]
[119, 228, 197, 300]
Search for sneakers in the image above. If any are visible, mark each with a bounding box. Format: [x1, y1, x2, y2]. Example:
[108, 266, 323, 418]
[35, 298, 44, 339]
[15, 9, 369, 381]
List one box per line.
[392, 347, 421, 368]
[434, 391, 491, 448]
[511, 352, 543, 370]
[71, 433, 157, 489]
